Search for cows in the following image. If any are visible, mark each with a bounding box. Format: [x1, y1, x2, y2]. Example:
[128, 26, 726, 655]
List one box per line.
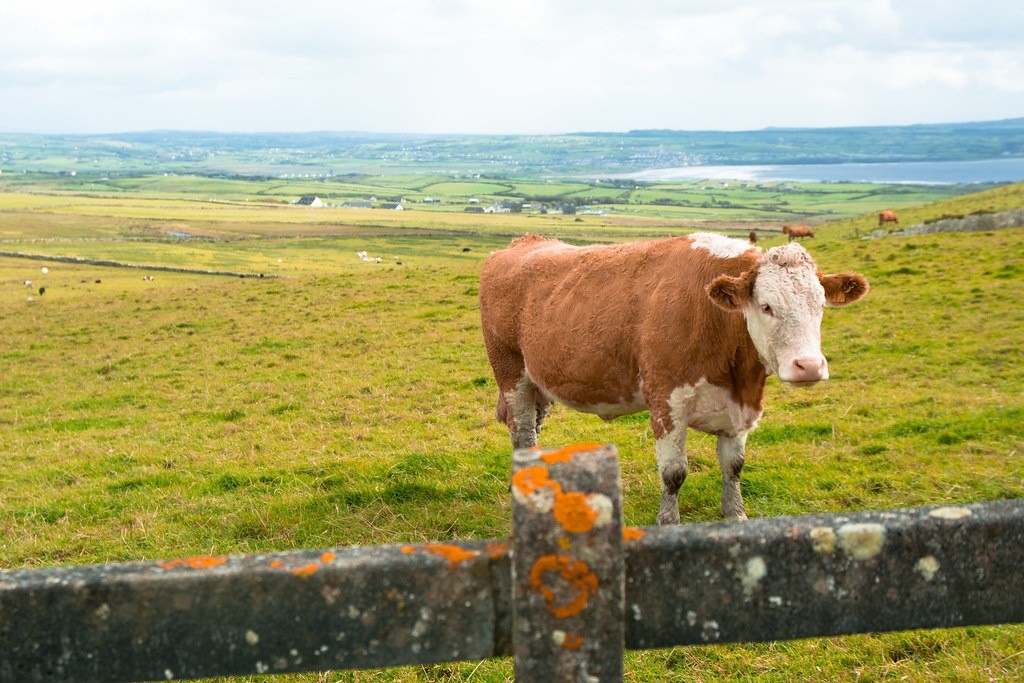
[782, 223, 816, 243]
[479, 229, 870, 522]
[879, 211, 900, 226]
